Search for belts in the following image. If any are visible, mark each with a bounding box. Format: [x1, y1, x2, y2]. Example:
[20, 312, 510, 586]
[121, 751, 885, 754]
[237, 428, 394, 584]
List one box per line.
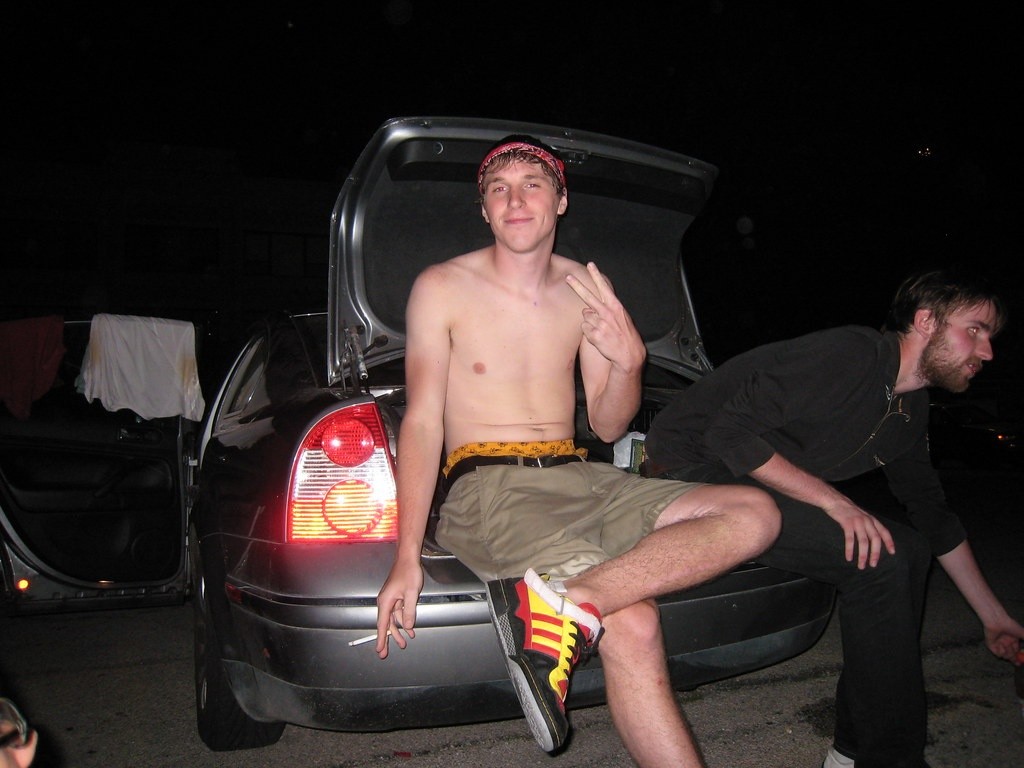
[443, 455, 588, 495]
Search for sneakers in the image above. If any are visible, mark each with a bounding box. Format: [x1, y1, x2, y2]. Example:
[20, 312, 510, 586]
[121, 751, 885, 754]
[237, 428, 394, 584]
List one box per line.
[484, 568, 603, 752]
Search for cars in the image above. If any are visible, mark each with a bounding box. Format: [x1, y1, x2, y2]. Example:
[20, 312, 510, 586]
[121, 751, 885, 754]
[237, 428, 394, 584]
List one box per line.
[0, 112, 845, 750]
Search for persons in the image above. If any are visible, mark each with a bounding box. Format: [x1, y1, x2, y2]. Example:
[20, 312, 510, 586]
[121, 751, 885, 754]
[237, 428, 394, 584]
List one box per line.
[646, 266, 1024, 768]
[373, 134, 783, 767]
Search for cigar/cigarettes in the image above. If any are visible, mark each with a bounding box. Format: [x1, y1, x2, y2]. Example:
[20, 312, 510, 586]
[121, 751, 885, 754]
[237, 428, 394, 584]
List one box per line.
[349, 631, 391, 647]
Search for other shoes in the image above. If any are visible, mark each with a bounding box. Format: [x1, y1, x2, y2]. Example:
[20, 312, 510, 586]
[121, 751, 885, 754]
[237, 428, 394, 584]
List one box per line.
[821, 750, 854, 768]
